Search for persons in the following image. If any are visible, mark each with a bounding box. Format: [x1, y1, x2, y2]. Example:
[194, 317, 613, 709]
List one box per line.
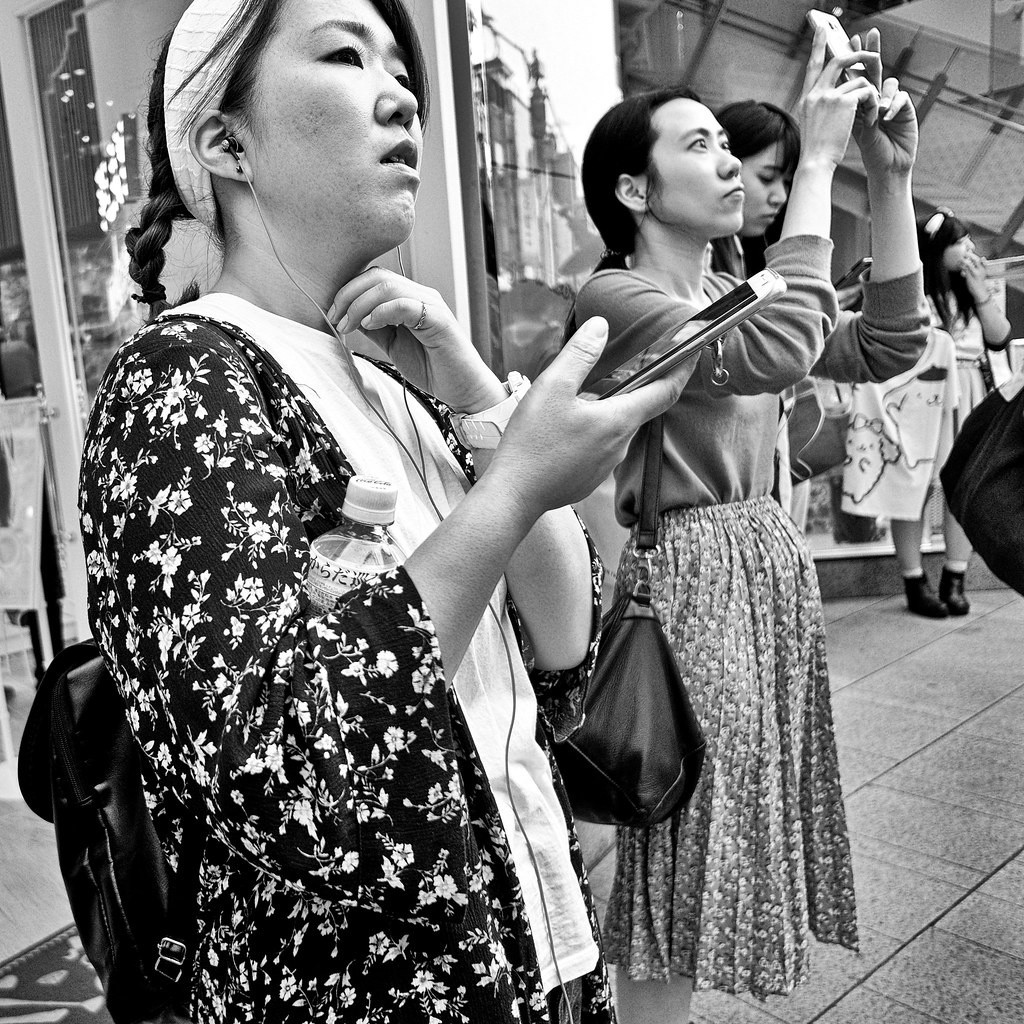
[888, 205, 1012, 619]
[711, 101, 802, 527]
[78, 0, 700, 1024]
[575, 24, 931, 1024]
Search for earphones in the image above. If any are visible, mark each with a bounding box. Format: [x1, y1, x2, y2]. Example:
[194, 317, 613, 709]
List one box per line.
[221, 133, 245, 160]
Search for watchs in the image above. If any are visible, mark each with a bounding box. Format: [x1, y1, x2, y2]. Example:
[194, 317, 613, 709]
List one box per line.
[450, 370, 531, 451]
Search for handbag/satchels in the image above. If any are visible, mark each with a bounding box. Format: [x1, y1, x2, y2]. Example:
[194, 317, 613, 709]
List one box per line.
[773, 373, 850, 485]
[549, 594, 706, 826]
[940, 367, 1024, 597]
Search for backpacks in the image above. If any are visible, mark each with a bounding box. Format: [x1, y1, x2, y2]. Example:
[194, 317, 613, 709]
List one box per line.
[18, 319, 305, 1024]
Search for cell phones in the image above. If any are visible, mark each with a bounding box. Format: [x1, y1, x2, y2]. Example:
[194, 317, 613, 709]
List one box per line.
[834, 257, 872, 290]
[805, 8, 883, 113]
[577, 265, 791, 403]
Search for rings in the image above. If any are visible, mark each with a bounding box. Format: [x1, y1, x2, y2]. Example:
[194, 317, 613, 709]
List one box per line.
[413, 301, 428, 330]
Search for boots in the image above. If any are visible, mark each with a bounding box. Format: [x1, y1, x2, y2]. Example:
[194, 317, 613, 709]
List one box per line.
[902, 571, 949, 618]
[937, 567, 970, 616]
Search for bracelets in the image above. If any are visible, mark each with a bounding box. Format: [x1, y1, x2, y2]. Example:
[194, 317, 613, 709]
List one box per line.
[976, 294, 992, 306]
